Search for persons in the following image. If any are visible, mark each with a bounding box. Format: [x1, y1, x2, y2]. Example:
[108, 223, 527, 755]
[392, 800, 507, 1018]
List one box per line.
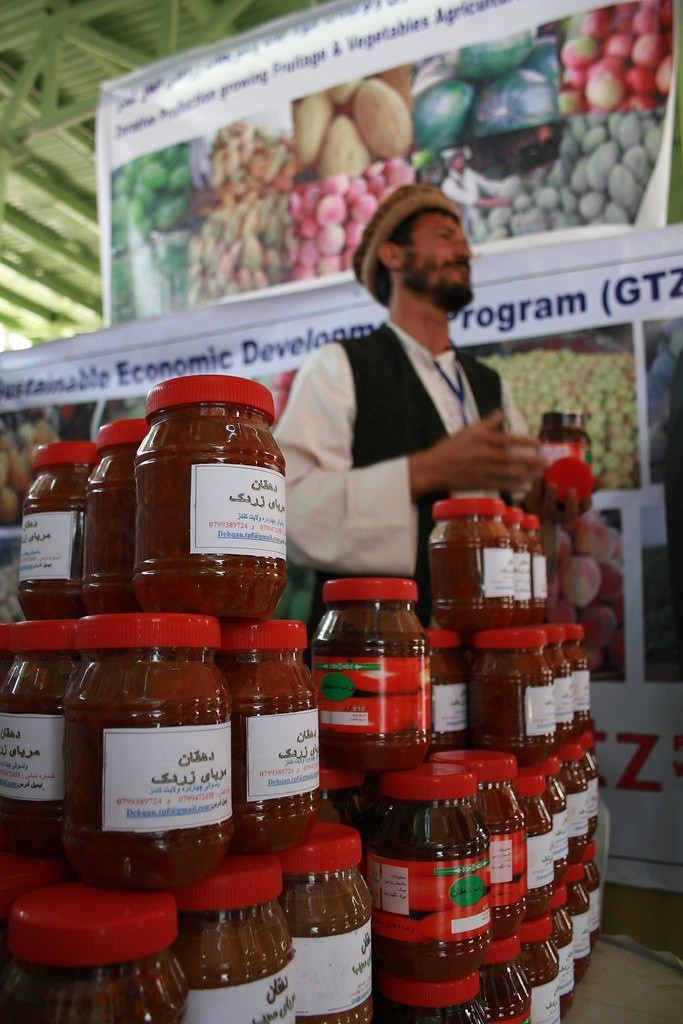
[270, 184, 601, 665]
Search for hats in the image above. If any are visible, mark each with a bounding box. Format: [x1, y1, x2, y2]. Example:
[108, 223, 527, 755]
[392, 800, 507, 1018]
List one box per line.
[351, 185, 462, 297]
